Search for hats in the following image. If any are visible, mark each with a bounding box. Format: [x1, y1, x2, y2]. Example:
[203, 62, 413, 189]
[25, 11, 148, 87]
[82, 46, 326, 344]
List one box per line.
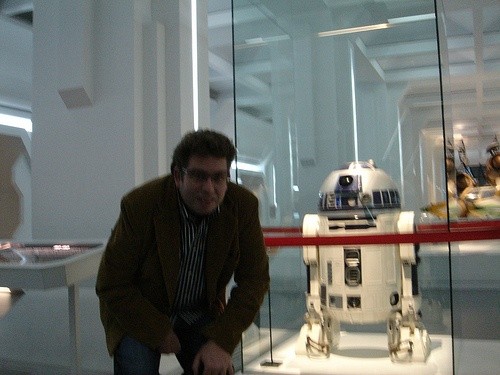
[456, 139, 466, 152]
[485, 132, 500, 156]
[445, 138, 453, 159]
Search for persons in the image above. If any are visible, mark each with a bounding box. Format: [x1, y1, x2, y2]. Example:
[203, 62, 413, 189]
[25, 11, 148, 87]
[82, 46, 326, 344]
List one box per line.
[94, 130, 272, 375]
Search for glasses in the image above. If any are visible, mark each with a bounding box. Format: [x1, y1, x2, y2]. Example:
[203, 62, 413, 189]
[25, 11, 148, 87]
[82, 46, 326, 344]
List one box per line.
[180, 167, 230, 182]
